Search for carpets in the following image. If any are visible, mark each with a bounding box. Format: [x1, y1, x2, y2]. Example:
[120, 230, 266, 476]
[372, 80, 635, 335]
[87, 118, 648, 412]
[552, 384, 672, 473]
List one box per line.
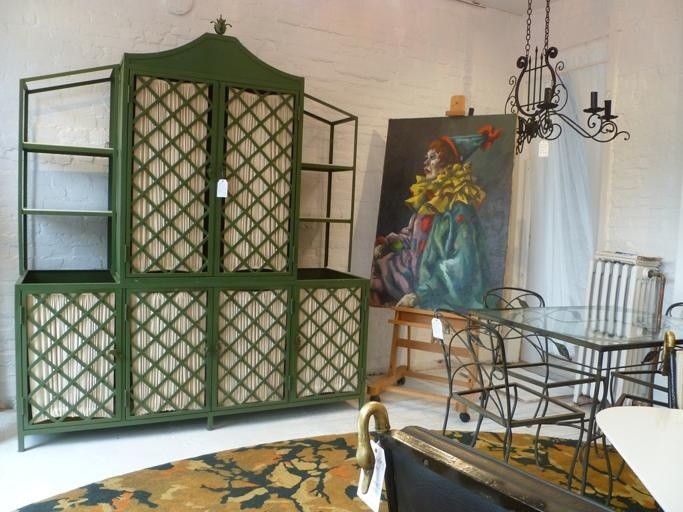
[16, 430, 661, 511]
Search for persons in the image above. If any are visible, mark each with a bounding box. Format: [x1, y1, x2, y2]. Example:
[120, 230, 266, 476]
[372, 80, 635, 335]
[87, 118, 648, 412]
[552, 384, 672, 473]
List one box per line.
[371, 135, 494, 314]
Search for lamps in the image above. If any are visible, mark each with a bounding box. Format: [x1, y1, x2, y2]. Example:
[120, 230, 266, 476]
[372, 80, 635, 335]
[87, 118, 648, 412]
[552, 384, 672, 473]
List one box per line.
[505, 0, 630, 155]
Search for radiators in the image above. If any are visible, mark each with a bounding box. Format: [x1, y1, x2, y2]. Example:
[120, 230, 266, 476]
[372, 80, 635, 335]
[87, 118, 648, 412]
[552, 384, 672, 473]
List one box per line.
[574, 259, 665, 405]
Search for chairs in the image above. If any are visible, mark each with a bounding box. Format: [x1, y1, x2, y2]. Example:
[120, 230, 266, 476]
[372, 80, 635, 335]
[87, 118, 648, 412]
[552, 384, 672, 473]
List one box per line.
[433, 305, 585, 492]
[610, 300, 683, 482]
[356, 397, 619, 512]
[482, 283, 608, 468]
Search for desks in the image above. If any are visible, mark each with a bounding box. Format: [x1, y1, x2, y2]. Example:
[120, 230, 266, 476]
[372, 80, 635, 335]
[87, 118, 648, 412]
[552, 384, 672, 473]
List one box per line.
[464, 303, 683, 496]
[595, 405, 681, 512]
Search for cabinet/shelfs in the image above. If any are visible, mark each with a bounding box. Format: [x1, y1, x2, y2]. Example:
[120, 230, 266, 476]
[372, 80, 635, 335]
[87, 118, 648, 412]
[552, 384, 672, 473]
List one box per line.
[12, 12, 370, 451]
